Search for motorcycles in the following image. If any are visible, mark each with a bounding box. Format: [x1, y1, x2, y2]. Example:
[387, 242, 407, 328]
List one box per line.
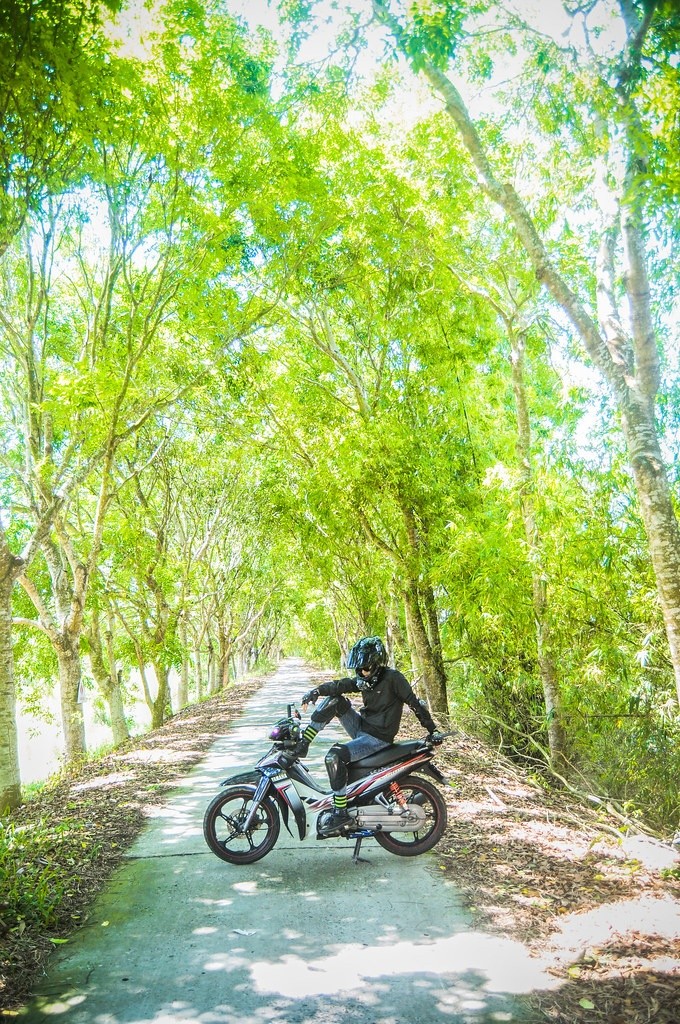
[201, 701, 458, 866]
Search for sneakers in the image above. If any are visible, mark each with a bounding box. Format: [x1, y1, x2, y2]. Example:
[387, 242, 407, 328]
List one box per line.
[276, 739, 308, 758]
[319, 809, 353, 834]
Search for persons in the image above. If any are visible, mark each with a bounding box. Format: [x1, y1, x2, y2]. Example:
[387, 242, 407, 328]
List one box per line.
[273, 634, 440, 834]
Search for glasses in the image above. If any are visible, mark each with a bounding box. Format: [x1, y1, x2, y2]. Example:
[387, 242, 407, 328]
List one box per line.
[357, 663, 374, 672]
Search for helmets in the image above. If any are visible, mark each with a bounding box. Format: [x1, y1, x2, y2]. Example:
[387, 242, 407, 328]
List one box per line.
[347, 636, 388, 690]
